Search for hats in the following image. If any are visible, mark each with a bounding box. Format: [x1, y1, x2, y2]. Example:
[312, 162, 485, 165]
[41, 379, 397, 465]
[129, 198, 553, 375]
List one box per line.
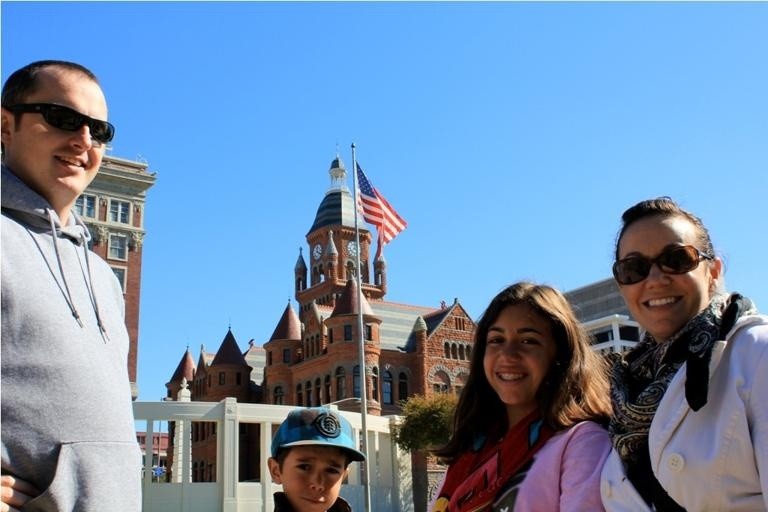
[271, 406, 366, 462]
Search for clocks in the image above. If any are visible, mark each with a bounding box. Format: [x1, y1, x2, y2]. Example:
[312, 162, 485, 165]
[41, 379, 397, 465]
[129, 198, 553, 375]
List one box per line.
[312, 242, 323, 261]
[346, 240, 362, 257]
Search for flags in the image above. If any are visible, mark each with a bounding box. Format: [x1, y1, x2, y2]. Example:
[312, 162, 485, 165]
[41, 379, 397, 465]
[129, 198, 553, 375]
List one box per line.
[355, 160, 408, 261]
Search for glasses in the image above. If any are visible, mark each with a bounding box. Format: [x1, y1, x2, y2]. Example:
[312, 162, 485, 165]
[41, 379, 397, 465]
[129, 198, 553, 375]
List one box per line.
[18, 103, 115, 144]
[612, 245, 711, 285]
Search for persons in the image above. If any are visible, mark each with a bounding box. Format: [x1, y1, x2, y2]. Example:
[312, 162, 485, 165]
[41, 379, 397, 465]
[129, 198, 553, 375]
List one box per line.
[267, 407, 366, 511]
[599, 195, 768, 511]
[0, 60, 144, 512]
[428, 280, 616, 511]
